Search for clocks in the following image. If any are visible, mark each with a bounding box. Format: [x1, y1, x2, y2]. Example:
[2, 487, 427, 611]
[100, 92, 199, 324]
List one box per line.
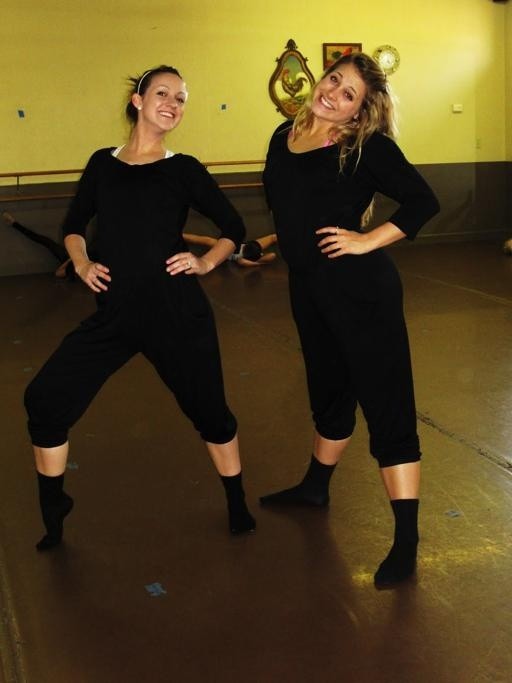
[373, 44, 401, 76]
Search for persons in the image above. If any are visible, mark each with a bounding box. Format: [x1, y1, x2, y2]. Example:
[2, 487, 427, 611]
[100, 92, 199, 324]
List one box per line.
[261, 50, 438, 588]
[0, 204, 82, 279]
[185, 229, 277, 267]
[23, 65, 260, 554]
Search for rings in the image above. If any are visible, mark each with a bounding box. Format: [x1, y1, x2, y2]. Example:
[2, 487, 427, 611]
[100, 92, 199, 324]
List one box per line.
[334, 225, 338, 234]
[187, 260, 193, 267]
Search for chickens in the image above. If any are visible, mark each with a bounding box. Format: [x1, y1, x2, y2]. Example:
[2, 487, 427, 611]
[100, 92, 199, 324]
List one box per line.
[282, 68, 308, 99]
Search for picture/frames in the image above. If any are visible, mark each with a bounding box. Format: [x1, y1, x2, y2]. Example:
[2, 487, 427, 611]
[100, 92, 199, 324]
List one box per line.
[267, 38, 318, 121]
[322, 41, 363, 70]
